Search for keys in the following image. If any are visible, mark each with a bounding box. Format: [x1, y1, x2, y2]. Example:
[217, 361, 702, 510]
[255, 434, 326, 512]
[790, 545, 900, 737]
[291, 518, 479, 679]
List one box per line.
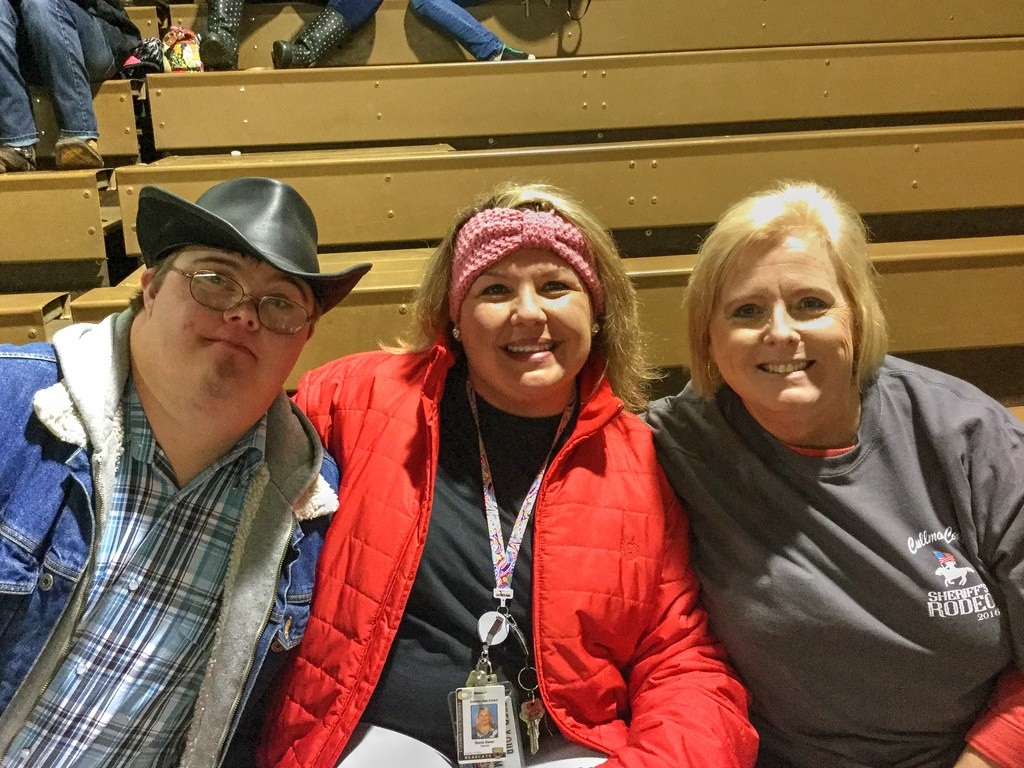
[517, 698, 546, 755]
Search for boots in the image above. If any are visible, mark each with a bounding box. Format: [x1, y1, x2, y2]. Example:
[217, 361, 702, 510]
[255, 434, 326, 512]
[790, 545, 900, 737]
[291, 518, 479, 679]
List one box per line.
[199, 0, 242, 70]
[272, 7, 352, 69]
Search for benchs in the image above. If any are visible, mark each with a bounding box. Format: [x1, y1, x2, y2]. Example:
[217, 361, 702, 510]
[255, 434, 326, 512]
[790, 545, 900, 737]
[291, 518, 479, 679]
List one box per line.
[0, 0, 1023, 421]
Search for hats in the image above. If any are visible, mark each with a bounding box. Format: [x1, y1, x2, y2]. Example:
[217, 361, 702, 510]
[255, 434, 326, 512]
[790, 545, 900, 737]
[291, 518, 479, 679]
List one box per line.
[136, 176, 372, 316]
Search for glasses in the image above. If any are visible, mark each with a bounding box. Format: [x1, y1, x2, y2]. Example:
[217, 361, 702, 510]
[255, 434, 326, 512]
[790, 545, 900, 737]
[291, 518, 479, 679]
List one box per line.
[168, 263, 314, 335]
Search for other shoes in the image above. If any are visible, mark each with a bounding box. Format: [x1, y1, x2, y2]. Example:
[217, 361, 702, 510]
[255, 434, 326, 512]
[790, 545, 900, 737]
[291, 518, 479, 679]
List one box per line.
[0, 145, 37, 173]
[55, 138, 104, 168]
[490, 43, 536, 61]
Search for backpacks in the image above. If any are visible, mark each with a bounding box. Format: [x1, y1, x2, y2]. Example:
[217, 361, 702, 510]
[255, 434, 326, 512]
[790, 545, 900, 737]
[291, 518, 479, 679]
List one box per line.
[122, 25, 206, 91]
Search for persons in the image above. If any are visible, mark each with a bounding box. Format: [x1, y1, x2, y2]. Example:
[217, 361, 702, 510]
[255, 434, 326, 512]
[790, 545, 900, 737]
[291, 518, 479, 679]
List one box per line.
[637, 179, 1024, 768]
[0, 178, 372, 768]
[199, 1, 536, 70]
[472, 705, 498, 739]
[252, 182, 760, 768]
[0, 1, 145, 175]
